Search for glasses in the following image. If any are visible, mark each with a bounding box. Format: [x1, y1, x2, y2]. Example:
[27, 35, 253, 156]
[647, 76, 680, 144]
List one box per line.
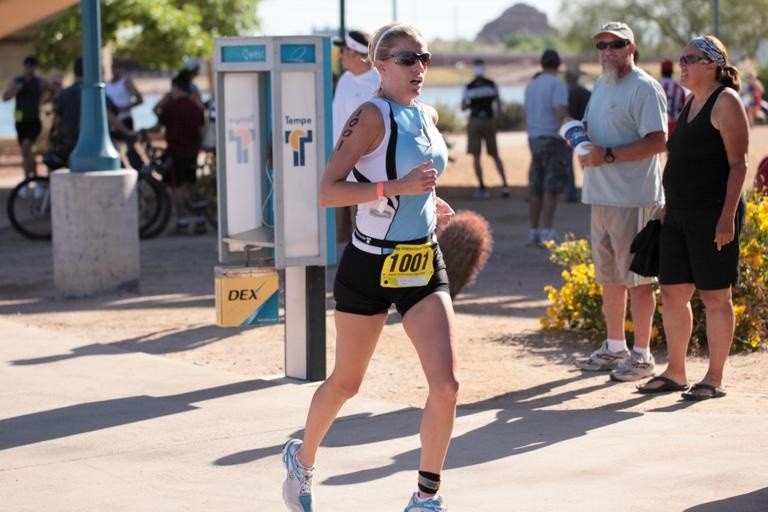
[596, 40, 630, 50]
[378, 51, 433, 66]
[680, 56, 711, 65]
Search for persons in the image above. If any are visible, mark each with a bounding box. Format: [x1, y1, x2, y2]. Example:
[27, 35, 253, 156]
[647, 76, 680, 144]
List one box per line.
[657, 60, 685, 139]
[522, 50, 571, 247]
[636, 33, 749, 400]
[559, 20, 670, 381]
[3, 57, 216, 237]
[461, 60, 510, 198]
[740, 73, 764, 127]
[332, 30, 381, 244]
[559, 67, 591, 204]
[283, 24, 460, 512]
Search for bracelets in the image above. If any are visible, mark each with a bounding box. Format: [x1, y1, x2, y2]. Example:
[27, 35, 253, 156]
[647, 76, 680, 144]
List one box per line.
[376, 182, 385, 202]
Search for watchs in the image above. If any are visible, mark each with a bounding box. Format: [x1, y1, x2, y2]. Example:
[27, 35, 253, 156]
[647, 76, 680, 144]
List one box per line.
[604, 148, 615, 164]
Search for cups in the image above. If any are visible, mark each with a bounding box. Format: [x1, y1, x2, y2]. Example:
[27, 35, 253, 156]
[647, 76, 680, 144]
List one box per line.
[558, 120, 592, 156]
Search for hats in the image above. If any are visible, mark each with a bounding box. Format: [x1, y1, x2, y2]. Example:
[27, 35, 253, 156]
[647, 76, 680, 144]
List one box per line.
[333, 32, 370, 55]
[591, 21, 635, 45]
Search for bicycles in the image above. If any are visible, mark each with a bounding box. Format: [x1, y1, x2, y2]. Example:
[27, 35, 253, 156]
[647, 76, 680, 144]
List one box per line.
[124, 129, 216, 241]
[6, 127, 162, 242]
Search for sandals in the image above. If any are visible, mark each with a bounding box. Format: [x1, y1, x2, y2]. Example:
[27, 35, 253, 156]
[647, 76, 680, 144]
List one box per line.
[681, 383, 726, 401]
[635, 377, 690, 393]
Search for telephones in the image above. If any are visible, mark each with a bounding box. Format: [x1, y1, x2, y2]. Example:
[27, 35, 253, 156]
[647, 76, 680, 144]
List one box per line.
[267, 137, 274, 171]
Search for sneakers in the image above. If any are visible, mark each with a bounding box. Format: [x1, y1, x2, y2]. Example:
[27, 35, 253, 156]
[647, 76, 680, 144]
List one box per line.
[529, 228, 558, 247]
[168, 219, 207, 237]
[404, 493, 446, 512]
[610, 350, 656, 381]
[281, 439, 315, 512]
[575, 339, 631, 370]
[18, 180, 44, 201]
[500, 187, 512, 198]
[473, 189, 489, 200]
